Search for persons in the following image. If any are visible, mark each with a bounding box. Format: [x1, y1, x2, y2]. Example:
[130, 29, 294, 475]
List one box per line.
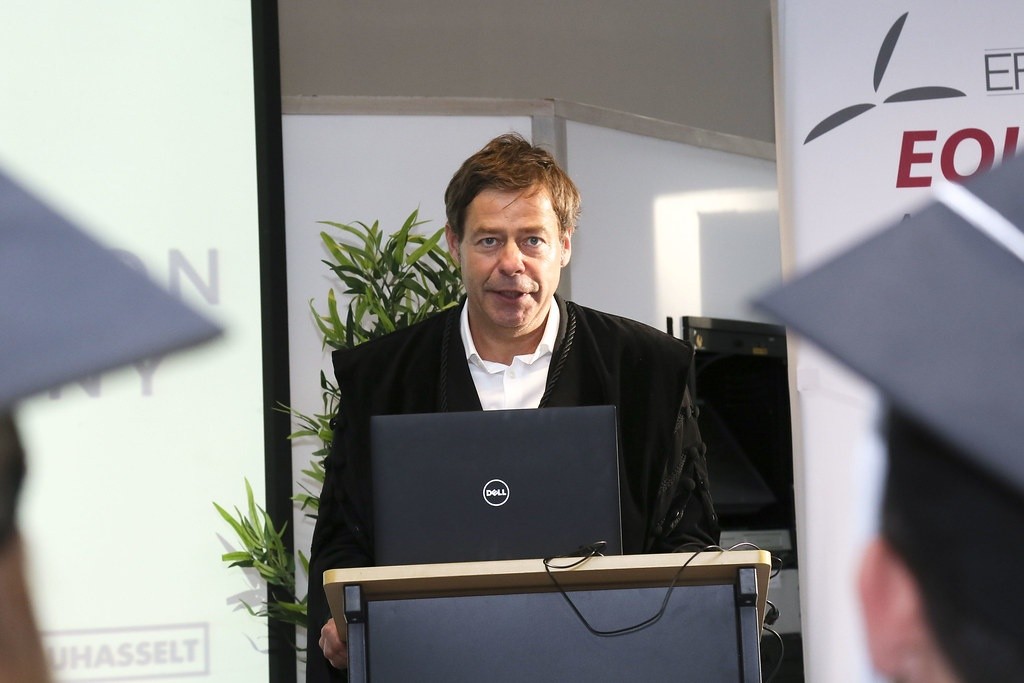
[302, 128, 726, 671]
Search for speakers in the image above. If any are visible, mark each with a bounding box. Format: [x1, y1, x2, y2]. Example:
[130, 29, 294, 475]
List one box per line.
[678, 318, 801, 568]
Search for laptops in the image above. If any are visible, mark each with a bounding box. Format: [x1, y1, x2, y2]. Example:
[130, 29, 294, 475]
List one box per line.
[371, 402, 624, 557]
